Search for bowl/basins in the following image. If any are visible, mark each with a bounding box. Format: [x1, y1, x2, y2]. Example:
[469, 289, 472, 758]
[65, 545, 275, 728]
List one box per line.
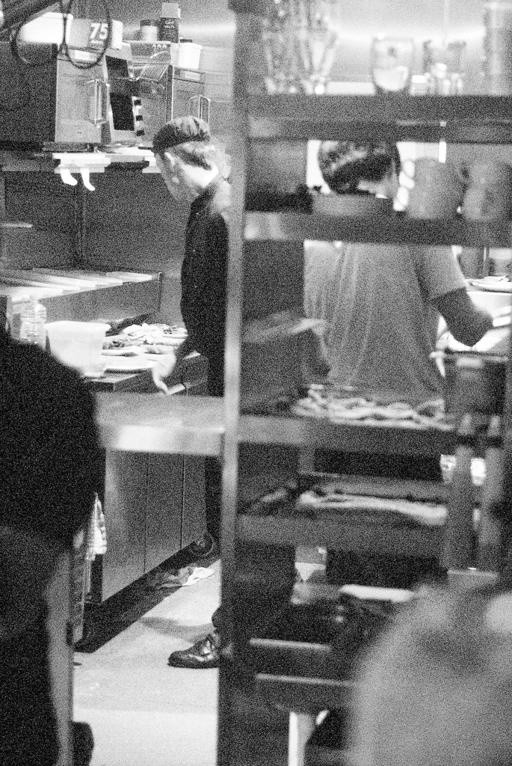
[44, 321, 111, 370]
[315, 195, 392, 217]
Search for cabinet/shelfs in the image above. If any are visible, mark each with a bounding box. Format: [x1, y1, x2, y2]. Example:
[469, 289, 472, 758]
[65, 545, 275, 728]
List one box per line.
[217, 0, 512, 766]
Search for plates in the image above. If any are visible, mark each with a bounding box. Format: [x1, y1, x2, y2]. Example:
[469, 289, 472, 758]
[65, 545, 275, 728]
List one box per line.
[104, 322, 187, 371]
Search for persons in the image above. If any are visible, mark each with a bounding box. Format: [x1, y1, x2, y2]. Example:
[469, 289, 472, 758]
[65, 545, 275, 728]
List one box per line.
[150, 114, 230, 669]
[303, 138, 494, 589]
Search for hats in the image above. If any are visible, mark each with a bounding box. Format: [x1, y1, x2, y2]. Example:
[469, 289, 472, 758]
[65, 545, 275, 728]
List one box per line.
[151, 115, 210, 152]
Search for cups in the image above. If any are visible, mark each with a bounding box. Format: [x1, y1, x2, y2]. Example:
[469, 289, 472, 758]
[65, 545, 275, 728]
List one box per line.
[139, 19, 162, 44]
[253, 0, 336, 98]
[456, 160, 510, 225]
[370, 35, 413, 94]
[479, 2, 512, 96]
[418, 41, 467, 95]
[399, 155, 463, 220]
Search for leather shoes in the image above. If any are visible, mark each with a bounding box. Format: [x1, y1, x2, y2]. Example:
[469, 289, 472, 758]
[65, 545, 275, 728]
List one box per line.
[166, 632, 226, 669]
